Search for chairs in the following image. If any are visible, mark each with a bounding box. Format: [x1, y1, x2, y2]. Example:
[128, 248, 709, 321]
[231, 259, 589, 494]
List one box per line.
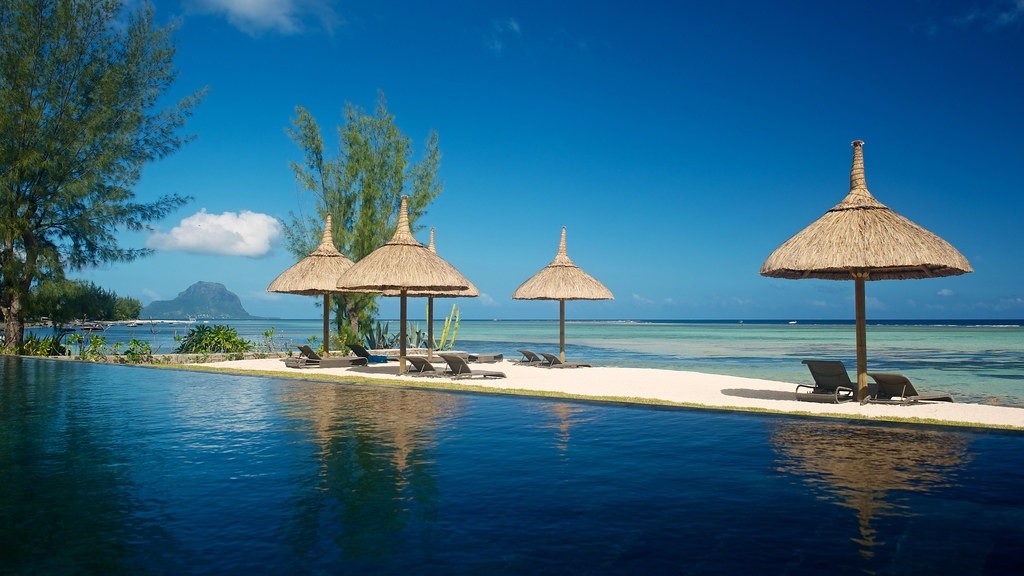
[866, 372, 954, 406]
[795, 360, 857, 404]
[293, 343, 322, 365]
[456, 352, 503, 363]
[401, 355, 454, 378]
[438, 354, 507, 380]
[536, 352, 592, 369]
[512, 348, 567, 367]
[346, 343, 400, 362]
